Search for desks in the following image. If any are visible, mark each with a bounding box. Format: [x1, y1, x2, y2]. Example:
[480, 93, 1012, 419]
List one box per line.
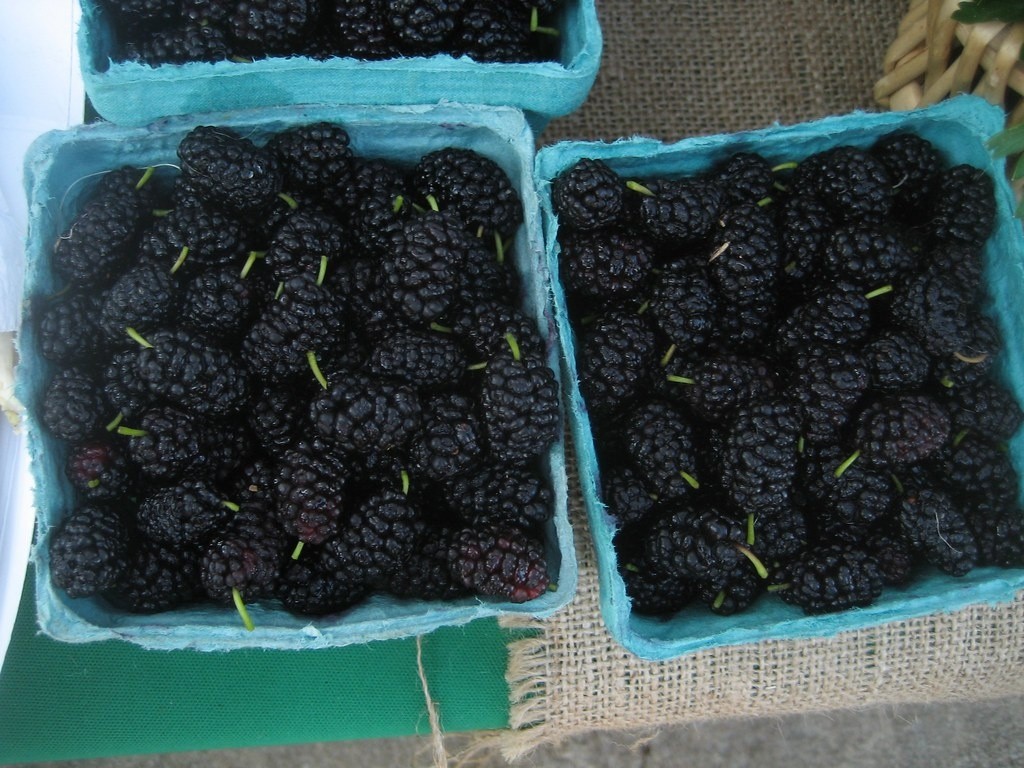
[0, 0, 1024, 765]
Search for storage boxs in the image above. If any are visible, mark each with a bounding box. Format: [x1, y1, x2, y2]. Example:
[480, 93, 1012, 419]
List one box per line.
[6, 101, 585, 655]
[72, 0, 606, 128]
[533, 91, 1024, 661]
